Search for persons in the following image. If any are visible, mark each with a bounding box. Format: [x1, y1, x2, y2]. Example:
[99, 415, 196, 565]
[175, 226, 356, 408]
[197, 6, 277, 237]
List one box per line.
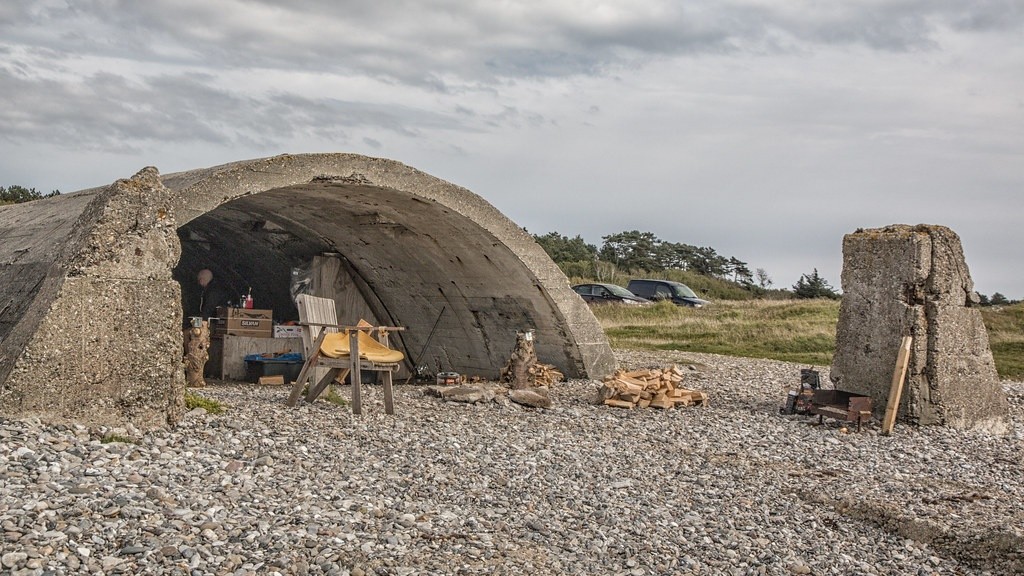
[196, 269, 229, 320]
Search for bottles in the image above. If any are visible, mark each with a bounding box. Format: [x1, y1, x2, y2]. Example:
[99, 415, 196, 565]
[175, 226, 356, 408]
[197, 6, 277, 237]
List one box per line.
[240, 295, 246, 308]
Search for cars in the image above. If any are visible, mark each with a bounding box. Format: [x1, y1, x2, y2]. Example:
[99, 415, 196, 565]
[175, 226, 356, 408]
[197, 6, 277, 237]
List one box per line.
[571, 283, 655, 307]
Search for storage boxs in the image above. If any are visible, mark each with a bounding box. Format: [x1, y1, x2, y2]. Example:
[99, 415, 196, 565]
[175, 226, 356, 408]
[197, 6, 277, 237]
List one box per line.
[216, 307, 274, 338]
[244, 359, 292, 384]
[289, 360, 309, 382]
[271, 325, 303, 338]
[345, 369, 376, 384]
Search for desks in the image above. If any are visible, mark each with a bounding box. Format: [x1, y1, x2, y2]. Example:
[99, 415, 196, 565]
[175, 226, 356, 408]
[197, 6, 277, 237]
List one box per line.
[203, 334, 305, 380]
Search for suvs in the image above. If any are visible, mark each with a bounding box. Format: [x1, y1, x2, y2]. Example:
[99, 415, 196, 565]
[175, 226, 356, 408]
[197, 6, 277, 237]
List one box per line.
[627, 279, 718, 308]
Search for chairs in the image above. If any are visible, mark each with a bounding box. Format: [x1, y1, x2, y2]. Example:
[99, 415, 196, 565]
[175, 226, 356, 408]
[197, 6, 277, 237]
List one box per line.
[285, 294, 405, 415]
